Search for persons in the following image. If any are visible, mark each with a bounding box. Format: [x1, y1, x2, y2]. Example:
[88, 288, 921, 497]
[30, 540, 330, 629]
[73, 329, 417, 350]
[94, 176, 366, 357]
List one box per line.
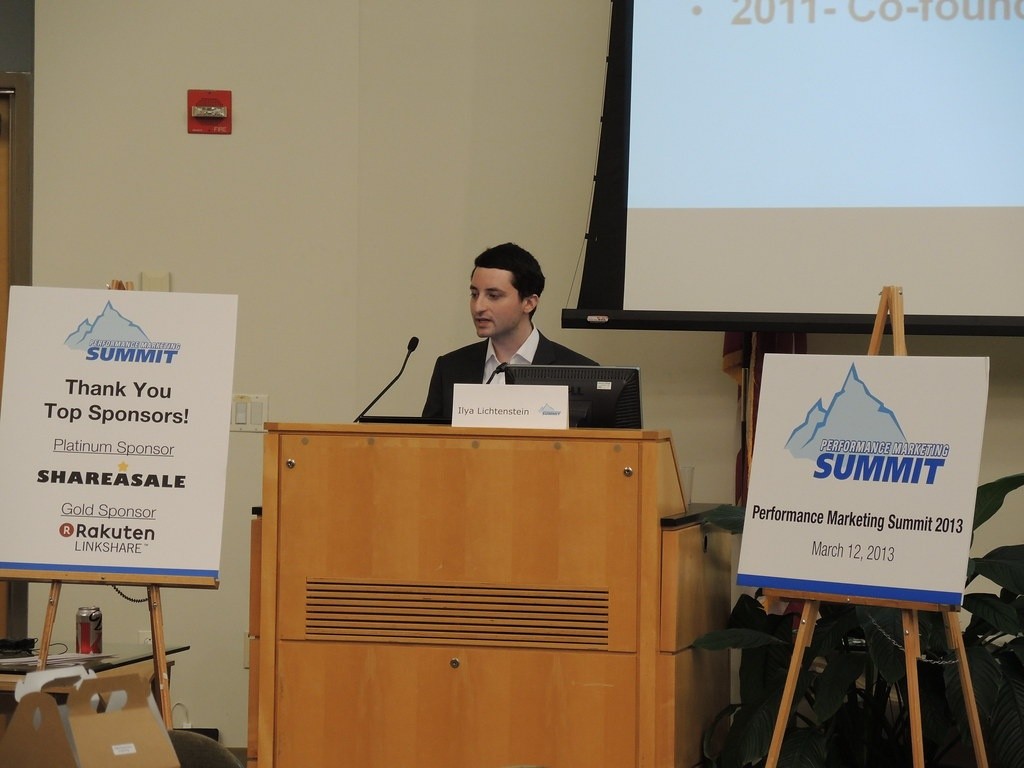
[421, 241, 600, 420]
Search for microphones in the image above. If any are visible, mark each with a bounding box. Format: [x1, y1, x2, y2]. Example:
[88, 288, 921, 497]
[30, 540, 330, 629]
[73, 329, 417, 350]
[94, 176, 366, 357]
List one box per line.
[485, 361, 509, 384]
[355, 337, 419, 423]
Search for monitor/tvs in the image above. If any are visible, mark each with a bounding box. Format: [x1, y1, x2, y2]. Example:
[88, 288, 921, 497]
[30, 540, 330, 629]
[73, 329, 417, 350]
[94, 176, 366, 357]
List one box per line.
[504, 364, 642, 428]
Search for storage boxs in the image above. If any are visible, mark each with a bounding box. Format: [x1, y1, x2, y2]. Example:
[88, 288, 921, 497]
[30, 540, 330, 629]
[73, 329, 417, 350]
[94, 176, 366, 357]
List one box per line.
[0, 665, 181, 768]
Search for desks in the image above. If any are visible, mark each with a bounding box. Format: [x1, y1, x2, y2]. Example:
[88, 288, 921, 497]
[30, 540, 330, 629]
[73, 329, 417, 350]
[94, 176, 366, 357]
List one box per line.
[1, 645, 190, 702]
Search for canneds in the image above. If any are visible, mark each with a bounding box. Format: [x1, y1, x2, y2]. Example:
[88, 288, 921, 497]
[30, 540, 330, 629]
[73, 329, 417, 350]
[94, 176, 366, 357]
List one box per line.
[76, 605, 102, 654]
